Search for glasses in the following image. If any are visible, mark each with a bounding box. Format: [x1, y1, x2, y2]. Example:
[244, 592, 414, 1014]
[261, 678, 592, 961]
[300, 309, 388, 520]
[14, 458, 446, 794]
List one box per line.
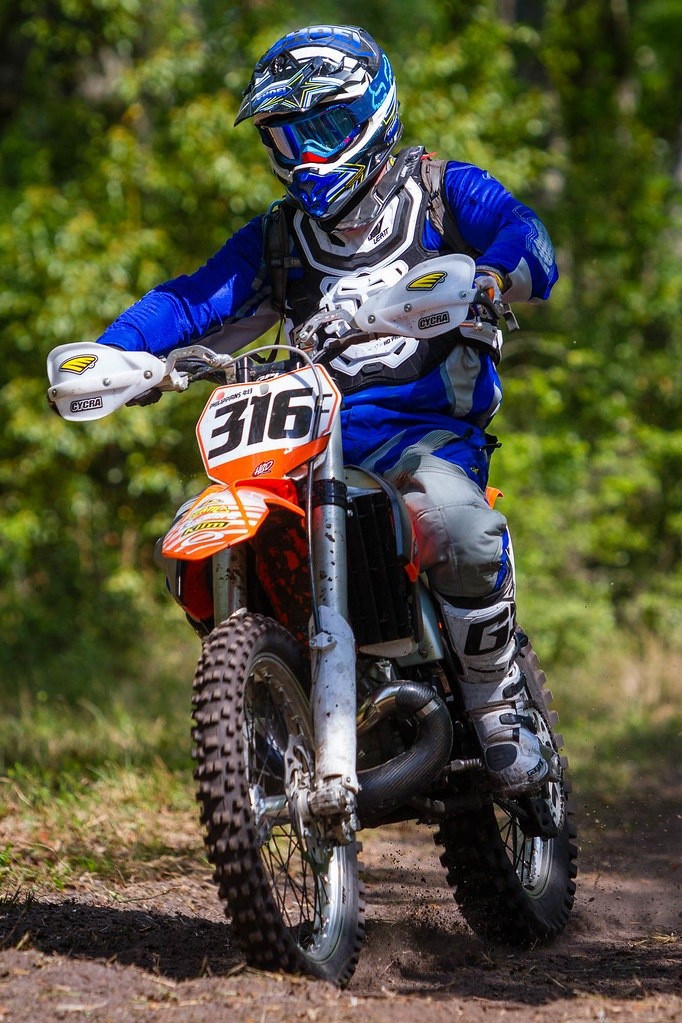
[258, 104, 361, 168]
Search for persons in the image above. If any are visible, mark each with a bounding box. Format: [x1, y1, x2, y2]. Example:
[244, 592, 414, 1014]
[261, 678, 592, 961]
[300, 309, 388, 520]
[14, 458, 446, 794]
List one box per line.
[96, 25, 561, 800]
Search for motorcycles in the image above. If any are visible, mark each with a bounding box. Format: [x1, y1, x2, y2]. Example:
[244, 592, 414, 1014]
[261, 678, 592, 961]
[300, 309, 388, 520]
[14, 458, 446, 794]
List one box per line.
[47, 253, 582, 990]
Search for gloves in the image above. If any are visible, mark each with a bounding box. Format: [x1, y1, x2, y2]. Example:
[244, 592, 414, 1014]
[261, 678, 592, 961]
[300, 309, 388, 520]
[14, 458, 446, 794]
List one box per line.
[135, 389, 163, 407]
[467, 271, 504, 323]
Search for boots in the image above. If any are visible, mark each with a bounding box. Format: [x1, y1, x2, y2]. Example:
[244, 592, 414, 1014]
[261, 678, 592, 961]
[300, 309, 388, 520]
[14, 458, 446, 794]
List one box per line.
[431, 566, 549, 794]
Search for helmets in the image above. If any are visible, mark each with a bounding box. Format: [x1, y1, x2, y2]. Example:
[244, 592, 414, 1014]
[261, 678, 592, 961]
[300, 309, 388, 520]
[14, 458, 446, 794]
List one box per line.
[233, 27, 398, 225]
